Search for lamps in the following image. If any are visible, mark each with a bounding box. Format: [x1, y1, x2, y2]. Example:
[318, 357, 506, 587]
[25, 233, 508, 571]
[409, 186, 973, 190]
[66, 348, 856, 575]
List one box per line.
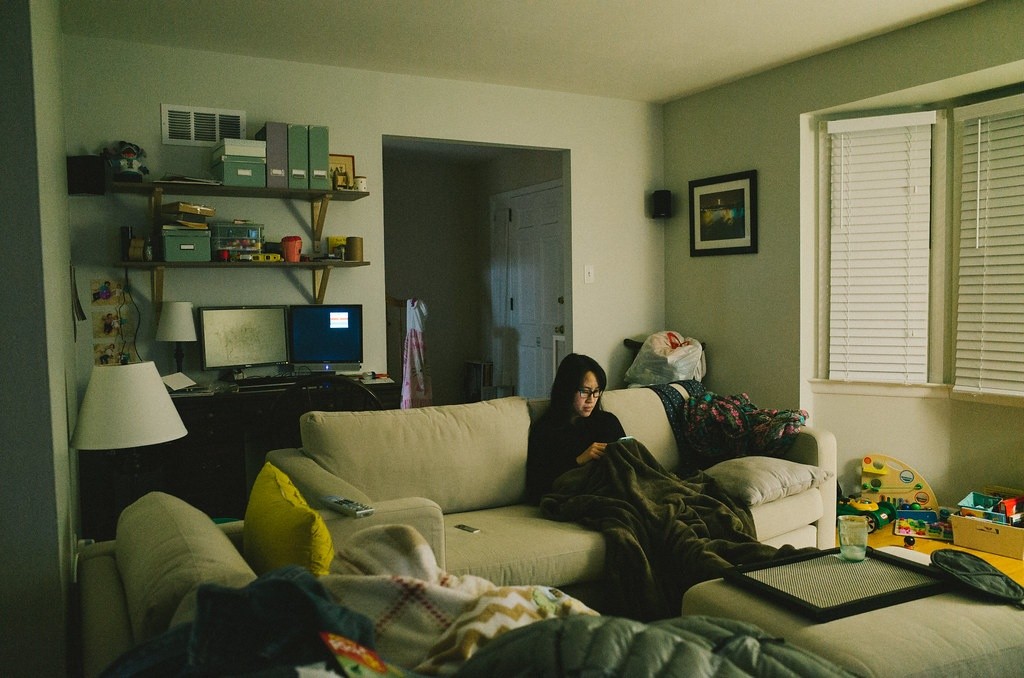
[155, 301, 206, 392]
[69, 361, 189, 502]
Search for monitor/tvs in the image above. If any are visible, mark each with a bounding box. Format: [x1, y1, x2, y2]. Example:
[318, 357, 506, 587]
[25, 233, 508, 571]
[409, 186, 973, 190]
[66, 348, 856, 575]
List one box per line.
[198, 304, 363, 372]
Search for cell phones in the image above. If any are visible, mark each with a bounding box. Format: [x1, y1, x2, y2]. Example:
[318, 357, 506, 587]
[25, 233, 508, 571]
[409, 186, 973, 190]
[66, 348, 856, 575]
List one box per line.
[618, 436, 633, 441]
[456, 525, 479, 533]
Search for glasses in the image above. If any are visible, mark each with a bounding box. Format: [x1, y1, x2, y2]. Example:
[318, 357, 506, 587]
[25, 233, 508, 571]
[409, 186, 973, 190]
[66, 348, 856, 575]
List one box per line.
[577, 387, 604, 399]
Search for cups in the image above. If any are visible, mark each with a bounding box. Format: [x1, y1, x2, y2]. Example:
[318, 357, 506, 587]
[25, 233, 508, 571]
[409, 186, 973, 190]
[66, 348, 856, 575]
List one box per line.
[837, 515, 869, 563]
[345, 237, 363, 262]
[353, 176, 367, 192]
[282, 236, 302, 262]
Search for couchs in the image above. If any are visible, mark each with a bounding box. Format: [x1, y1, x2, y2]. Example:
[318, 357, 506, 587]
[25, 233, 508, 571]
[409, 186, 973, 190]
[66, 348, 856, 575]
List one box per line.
[679, 577, 1024, 678]
[263, 388, 837, 589]
[76, 496, 446, 678]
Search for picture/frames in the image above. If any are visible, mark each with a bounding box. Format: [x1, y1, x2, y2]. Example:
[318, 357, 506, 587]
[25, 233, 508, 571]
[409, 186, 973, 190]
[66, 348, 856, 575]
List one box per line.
[329, 154, 355, 190]
[689, 169, 759, 257]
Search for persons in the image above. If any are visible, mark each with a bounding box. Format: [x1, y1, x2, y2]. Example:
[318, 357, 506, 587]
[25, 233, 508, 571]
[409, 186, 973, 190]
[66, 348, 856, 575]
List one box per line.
[99, 281, 122, 366]
[528, 352, 627, 501]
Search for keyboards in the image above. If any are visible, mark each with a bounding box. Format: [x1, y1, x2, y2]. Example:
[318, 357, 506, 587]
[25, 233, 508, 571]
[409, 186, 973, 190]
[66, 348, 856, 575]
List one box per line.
[234, 375, 309, 388]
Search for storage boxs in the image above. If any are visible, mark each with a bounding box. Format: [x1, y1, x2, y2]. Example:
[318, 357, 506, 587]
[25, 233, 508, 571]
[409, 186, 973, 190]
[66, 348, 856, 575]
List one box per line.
[210, 156, 266, 188]
[213, 138, 267, 160]
[152, 230, 211, 262]
[946, 511, 1024, 561]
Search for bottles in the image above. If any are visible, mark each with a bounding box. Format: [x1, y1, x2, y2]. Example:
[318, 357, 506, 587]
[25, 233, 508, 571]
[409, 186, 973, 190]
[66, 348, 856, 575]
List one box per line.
[142, 231, 153, 261]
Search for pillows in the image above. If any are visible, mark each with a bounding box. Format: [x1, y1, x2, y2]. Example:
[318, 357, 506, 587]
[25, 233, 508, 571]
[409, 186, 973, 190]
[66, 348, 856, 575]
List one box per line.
[696, 455, 834, 509]
[114, 491, 258, 647]
[243, 461, 336, 578]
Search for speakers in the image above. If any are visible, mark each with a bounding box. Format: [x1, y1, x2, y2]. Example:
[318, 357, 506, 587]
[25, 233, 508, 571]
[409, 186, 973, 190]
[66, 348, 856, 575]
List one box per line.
[652, 190, 673, 219]
[68, 155, 105, 195]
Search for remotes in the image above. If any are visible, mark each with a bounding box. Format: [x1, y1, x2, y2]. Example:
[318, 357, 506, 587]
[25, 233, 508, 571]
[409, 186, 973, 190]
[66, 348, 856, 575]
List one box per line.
[319, 495, 374, 518]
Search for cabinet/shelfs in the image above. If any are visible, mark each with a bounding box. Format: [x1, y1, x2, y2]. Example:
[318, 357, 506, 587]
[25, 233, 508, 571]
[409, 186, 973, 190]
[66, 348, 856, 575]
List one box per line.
[112, 182, 371, 326]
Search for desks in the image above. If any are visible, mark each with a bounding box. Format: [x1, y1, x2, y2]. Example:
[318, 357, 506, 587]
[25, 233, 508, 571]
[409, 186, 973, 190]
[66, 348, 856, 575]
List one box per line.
[77, 375, 403, 539]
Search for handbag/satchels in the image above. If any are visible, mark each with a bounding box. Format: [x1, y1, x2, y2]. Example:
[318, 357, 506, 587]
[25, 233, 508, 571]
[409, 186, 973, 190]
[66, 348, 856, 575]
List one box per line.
[625, 331, 703, 384]
